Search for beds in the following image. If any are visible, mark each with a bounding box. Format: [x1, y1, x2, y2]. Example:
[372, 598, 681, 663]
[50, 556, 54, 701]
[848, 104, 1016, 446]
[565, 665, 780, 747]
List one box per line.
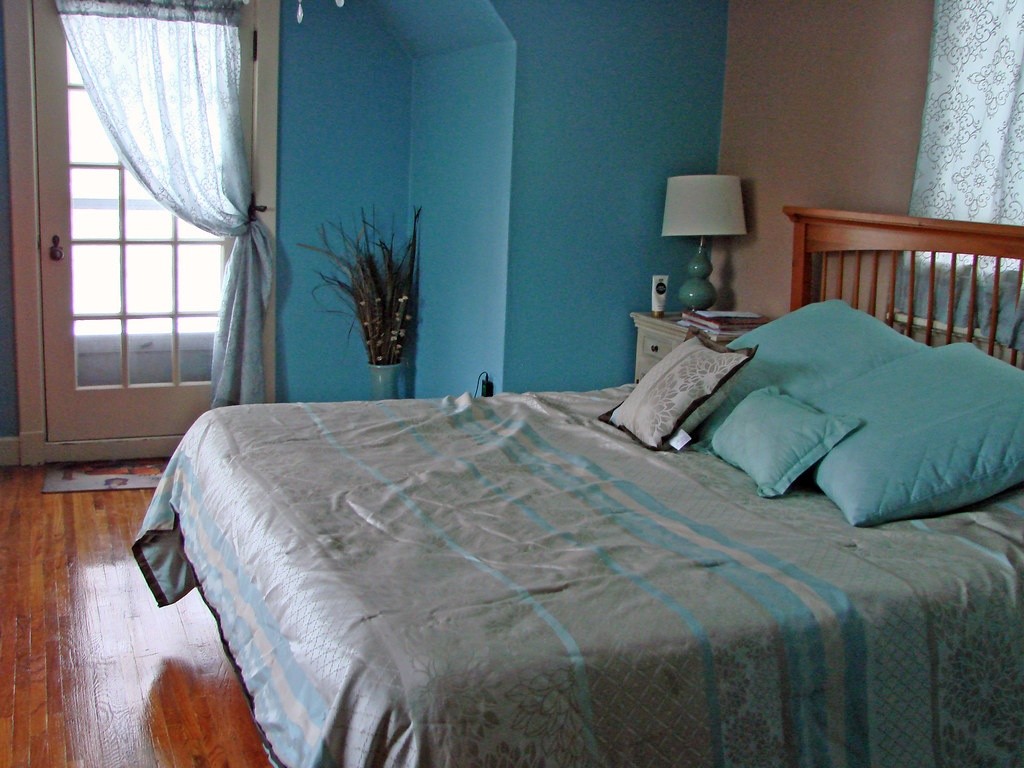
[131, 207, 1024, 768]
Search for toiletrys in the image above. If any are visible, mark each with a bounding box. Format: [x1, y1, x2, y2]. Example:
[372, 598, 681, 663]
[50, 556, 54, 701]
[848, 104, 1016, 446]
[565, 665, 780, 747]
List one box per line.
[651, 274, 669, 318]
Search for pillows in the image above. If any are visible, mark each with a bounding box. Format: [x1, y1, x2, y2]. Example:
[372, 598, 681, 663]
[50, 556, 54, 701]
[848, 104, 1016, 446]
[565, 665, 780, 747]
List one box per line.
[802, 343, 1024, 526]
[689, 385, 861, 498]
[597, 326, 759, 451]
[687, 298, 933, 443]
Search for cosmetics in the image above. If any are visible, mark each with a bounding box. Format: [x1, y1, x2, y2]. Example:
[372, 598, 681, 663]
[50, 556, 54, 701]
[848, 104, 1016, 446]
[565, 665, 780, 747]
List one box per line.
[652, 275, 669, 319]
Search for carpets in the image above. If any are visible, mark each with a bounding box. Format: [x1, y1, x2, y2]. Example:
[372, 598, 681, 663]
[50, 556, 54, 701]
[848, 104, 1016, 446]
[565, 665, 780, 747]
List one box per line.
[40, 456, 171, 493]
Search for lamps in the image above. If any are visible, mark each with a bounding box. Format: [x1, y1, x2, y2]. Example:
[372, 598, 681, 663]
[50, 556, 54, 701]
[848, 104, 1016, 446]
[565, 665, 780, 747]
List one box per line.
[661, 174, 747, 309]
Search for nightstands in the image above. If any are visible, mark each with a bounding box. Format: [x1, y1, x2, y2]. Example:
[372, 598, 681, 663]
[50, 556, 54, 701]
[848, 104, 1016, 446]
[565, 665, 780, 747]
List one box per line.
[629, 311, 749, 385]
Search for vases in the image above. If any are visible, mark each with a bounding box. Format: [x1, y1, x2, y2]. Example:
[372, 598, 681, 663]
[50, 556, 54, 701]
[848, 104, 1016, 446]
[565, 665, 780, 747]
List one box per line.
[367, 362, 402, 400]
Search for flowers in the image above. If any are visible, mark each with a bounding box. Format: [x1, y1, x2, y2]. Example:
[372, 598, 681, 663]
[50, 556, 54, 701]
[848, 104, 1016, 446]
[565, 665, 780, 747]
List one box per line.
[295, 204, 422, 366]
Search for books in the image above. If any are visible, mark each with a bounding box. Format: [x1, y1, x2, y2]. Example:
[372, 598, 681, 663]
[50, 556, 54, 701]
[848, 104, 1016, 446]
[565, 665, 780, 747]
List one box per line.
[678, 311, 773, 341]
